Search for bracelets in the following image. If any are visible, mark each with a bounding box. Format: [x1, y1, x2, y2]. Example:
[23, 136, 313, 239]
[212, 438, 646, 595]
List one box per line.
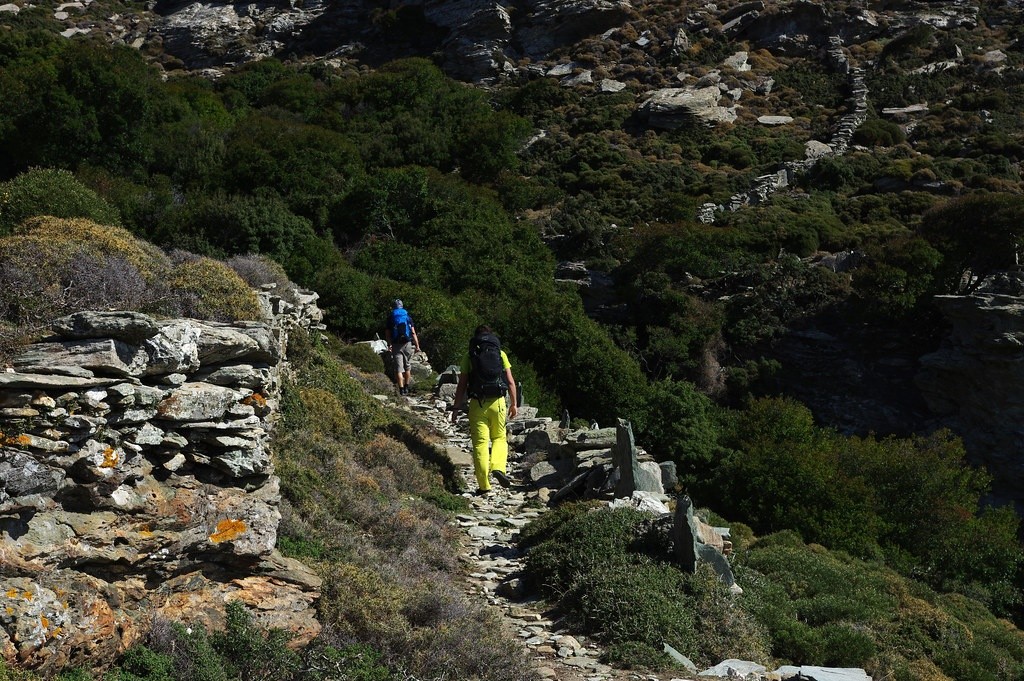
[453, 407, 458, 409]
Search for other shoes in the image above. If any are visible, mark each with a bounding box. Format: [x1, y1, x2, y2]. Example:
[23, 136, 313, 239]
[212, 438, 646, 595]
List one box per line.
[475, 488, 489, 496]
[492, 469, 510, 488]
[400, 387, 409, 395]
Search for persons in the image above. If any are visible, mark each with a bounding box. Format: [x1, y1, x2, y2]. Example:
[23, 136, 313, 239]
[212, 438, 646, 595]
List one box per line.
[452, 324, 517, 495]
[386, 299, 421, 394]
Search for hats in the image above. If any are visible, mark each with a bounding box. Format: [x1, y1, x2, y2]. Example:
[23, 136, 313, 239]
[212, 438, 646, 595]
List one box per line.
[393, 298, 404, 309]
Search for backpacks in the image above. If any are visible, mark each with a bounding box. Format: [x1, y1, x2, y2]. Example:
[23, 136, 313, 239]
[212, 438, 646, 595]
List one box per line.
[388, 307, 414, 344]
[465, 331, 509, 408]
